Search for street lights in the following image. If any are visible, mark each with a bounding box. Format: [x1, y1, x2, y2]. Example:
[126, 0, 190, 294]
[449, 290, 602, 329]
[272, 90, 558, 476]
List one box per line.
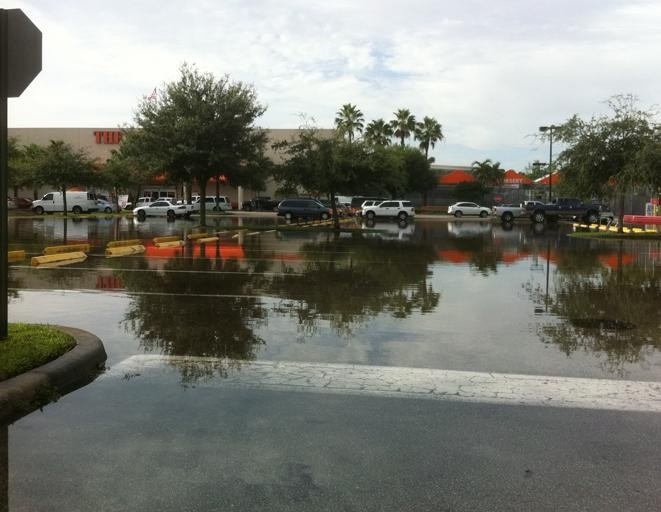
[533, 163, 550, 167]
[533, 226, 559, 315]
[538, 125, 563, 200]
